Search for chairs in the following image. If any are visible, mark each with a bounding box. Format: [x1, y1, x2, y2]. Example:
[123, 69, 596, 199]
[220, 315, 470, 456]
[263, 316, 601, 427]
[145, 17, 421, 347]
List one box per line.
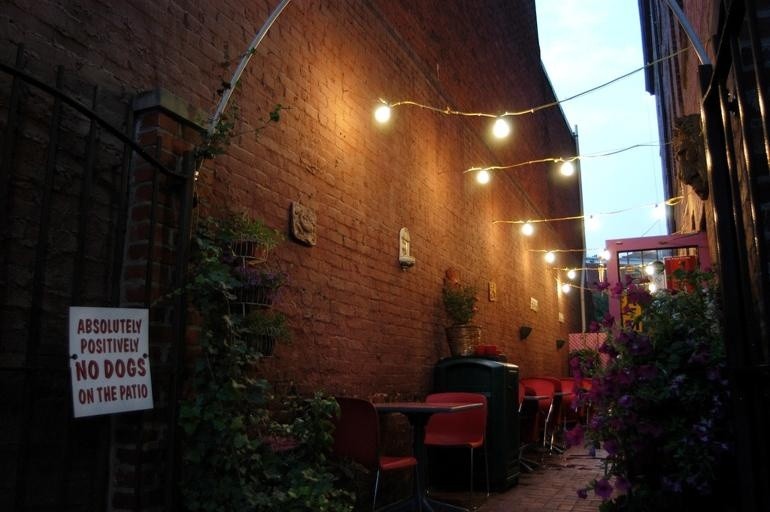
[320, 374, 594, 511]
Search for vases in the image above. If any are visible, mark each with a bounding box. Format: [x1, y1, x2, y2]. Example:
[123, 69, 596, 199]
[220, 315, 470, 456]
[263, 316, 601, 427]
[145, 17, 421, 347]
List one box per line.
[243, 335, 277, 357]
[445, 325, 482, 357]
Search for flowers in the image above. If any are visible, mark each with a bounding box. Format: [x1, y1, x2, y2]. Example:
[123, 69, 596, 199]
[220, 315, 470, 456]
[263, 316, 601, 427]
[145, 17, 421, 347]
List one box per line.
[441, 265, 482, 325]
[565, 261, 730, 509]
[218, 208, 295, 346]
